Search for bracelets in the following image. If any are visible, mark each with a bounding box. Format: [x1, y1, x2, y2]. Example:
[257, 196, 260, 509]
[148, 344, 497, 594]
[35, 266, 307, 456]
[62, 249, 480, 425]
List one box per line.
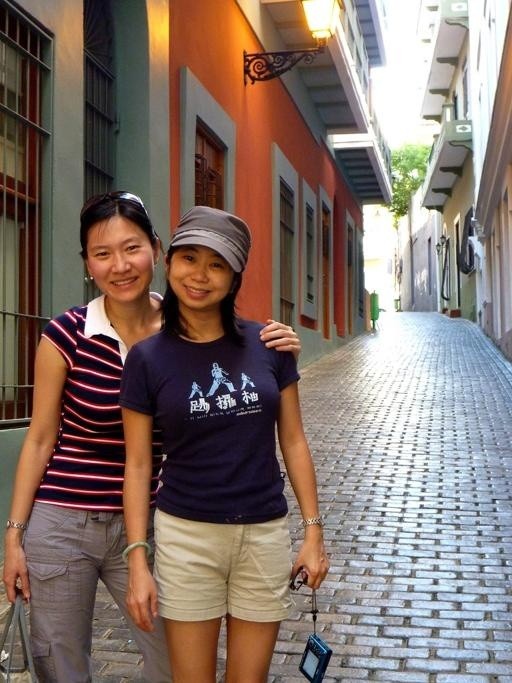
[301, 512, 324, 527]
[122, 540, 152, 562]
[5, 519, 29, 531]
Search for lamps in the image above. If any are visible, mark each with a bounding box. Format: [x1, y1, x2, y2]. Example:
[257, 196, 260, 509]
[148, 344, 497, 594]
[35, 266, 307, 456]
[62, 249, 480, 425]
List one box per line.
[435, 234, 450, 255]
[242, 0, 351, 97]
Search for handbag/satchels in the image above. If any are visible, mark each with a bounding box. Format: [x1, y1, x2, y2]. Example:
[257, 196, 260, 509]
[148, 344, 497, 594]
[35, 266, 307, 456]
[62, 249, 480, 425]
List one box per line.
[0, 591, 37, 682]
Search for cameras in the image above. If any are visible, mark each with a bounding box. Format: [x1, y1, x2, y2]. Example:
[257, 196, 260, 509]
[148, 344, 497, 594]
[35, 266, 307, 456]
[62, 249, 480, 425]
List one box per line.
[298, 634, 333, 683]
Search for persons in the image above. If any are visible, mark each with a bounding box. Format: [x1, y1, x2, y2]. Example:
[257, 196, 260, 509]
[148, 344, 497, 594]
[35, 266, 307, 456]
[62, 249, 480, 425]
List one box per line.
[117, 205, 332, 683]
[0, 191, 304, 683]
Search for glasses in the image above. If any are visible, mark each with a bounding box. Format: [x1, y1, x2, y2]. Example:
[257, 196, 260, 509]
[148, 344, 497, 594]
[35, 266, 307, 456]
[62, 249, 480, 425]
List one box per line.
[78, 191, 145, 218]
[287, 567, 308, 592]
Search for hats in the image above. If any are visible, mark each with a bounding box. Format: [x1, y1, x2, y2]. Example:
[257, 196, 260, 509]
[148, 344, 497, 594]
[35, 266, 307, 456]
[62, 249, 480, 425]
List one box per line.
[172, 206, 252, 275]
